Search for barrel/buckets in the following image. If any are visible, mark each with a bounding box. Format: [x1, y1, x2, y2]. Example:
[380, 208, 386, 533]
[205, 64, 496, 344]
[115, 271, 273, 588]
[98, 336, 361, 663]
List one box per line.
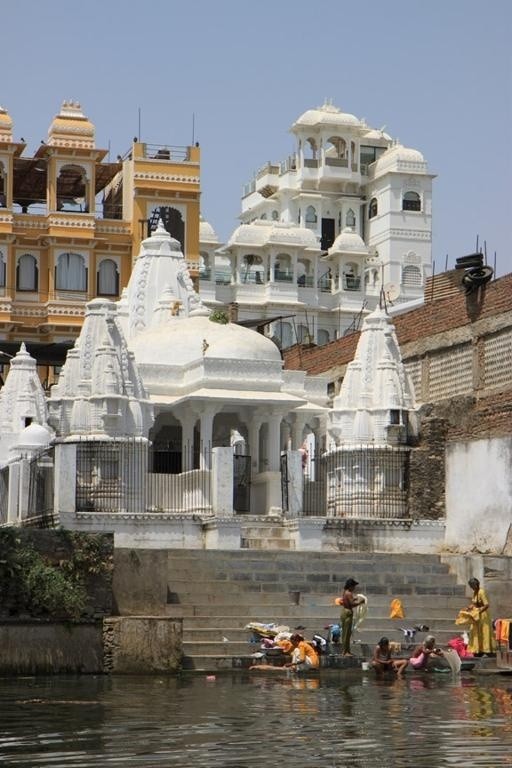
[288, 591, 299, 606]
[361, 662, 370, 671]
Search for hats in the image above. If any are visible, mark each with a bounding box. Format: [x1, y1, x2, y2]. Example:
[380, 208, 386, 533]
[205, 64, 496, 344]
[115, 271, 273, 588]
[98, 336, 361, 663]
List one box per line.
[344, 579, 359, 590]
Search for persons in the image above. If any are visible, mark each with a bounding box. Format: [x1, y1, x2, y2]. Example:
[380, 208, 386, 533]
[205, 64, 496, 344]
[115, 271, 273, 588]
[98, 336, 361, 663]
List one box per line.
[460, 578, 498, 654]
[372, 637, 408, 678]
[284, 633, 319, 673]
[340, 578, 365, 657]
[410, 636, 444, 672]
